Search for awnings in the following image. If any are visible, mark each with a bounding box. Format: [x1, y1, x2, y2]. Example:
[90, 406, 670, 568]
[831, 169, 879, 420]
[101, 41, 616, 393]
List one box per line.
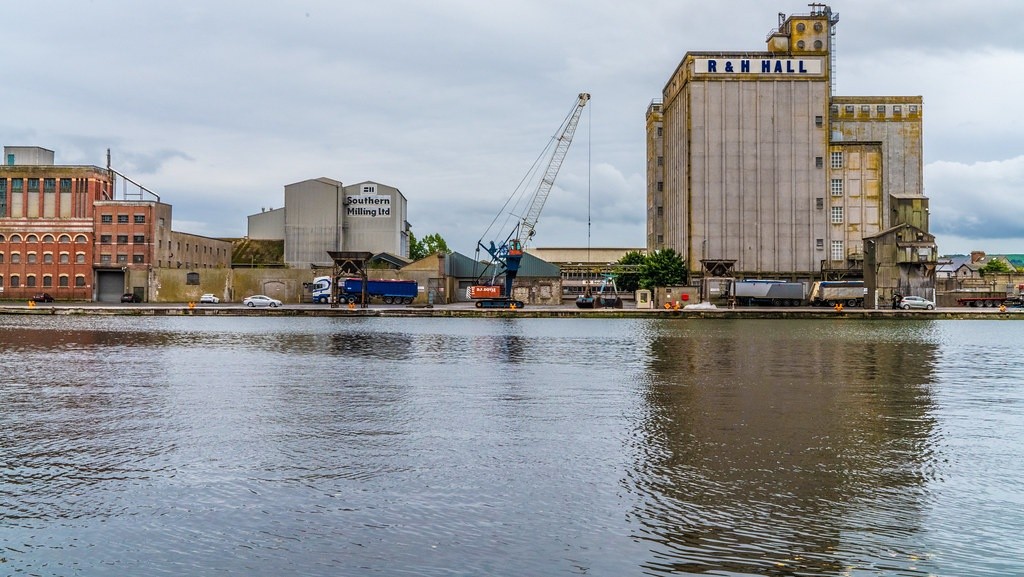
[897, 259, 954, 273]
[897, 240, 937, 248]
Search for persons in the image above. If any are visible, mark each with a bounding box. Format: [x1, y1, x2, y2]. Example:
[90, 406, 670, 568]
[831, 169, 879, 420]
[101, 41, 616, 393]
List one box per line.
[891, 292, 903, 310]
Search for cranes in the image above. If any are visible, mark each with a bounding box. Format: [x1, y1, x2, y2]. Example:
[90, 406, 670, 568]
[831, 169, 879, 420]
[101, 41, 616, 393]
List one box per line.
[465, 92, 596, 309]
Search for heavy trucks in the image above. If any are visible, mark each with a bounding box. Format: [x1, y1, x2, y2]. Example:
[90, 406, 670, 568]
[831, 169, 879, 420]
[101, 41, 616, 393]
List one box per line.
[311, 276, 419, 305]
[955, 283, 1024, 308]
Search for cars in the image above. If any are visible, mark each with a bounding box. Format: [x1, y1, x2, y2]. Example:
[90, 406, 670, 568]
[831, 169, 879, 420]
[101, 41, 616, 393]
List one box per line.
[32, 292, 55, 303]
[121, 293, 141, 304]
[200, 293, 220, 303]
[243, 294, 283, 308]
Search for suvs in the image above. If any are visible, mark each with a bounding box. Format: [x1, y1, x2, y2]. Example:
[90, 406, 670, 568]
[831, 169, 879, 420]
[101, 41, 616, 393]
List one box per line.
[899, 296, 936, 310]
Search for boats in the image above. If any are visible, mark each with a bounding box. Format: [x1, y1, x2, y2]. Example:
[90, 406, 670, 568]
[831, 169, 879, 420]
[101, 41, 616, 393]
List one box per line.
[575, 269, 623, 309]
